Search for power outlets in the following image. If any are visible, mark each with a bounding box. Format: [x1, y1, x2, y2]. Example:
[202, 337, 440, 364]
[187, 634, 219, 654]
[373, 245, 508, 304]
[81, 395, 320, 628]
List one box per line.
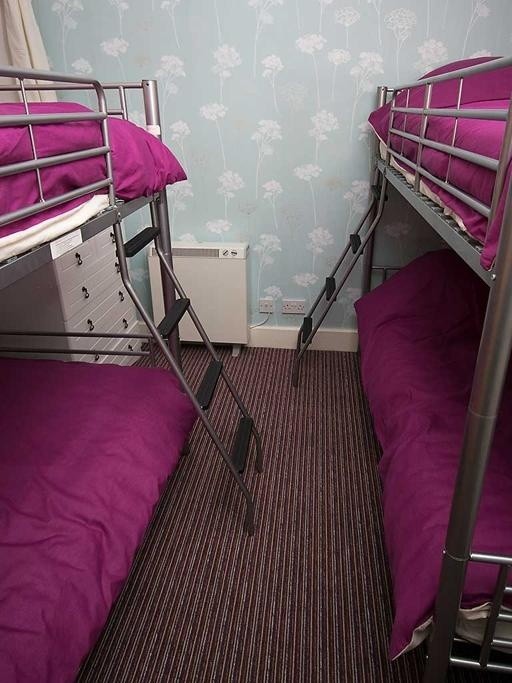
[281, 299, 306, 314]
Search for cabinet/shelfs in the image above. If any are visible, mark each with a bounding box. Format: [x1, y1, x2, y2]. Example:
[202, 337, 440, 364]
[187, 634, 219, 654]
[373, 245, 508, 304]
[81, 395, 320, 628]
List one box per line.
[0, 217, 142, 366]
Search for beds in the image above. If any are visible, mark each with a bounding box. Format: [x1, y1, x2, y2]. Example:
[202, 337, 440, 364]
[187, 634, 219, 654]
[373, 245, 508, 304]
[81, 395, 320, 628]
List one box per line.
[0, 68, 264, 683]
[292, 53, 512, 683]
